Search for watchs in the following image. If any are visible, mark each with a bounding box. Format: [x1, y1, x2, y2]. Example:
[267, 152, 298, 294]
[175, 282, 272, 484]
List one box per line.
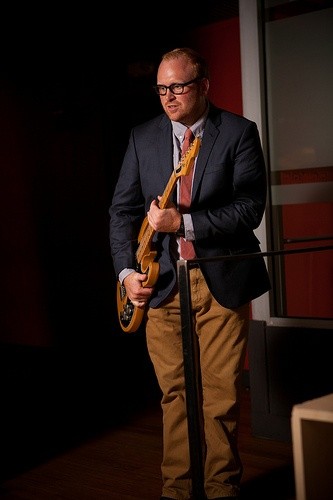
[175, 216, 185, 239]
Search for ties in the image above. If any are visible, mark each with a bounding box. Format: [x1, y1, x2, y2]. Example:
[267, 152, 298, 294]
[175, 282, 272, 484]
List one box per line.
[179, 129, 196, 260]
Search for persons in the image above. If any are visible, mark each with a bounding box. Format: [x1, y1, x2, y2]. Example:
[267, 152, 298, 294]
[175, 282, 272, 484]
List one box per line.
[107, 48, 272, 500]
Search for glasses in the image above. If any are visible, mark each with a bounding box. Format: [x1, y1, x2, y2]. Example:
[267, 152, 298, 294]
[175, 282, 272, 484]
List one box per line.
[153, 77, 198, 95]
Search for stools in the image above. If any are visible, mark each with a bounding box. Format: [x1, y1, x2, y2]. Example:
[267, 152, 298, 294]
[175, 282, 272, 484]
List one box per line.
[291, 393, 333, 500]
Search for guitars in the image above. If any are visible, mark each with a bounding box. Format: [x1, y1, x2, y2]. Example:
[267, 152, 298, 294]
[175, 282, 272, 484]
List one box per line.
[115, 136, 202, 332]
